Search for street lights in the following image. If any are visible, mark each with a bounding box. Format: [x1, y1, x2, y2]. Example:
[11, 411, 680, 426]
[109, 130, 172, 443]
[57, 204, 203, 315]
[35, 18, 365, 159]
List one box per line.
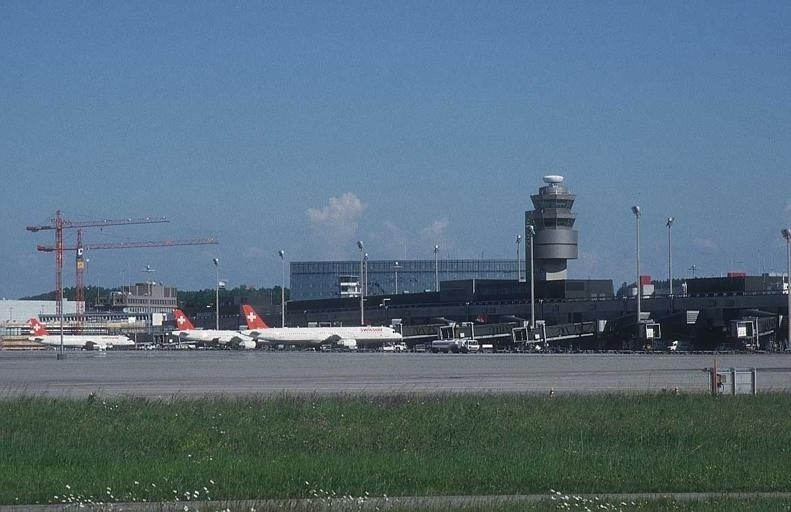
[630, 204, 644, 321]
[364, 253, 369, 296]
[211, 257, 223, 330]
[665, 211, 677, 297]
[140, 263, 159, 335]
[689, 263, 697, 279]
[527, 224, 539, 327]
[780, 225, 791, 356]
[514, 233, 523, 282]
[278, 249, 289, 327]
[356, 238, 367, 326]
[432, 243, 440, 292]
[392, 261, 400, 293]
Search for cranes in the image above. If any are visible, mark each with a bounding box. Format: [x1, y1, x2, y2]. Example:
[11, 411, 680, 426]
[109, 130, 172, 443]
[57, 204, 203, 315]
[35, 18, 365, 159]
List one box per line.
[24, 209, 170, 323]
[37, 229, 221, 338]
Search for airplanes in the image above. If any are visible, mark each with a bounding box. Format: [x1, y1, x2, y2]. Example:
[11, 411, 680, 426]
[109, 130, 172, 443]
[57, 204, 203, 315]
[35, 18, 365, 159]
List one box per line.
[238, 303, 404, 353]
[171, 309, 286, 350]
[27, 317, 136, 351]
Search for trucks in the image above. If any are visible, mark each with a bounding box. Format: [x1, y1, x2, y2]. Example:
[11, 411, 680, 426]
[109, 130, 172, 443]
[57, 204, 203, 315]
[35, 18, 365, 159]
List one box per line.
[667, 339, 698, 352]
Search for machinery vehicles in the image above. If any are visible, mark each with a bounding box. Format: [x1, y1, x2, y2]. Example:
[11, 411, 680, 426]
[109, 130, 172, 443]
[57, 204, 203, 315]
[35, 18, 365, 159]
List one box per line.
[431, 337, 481, 354]
[81, 340, 109, 351]
[377, 341, 430, 353]
[133, 340, 193, 352]
